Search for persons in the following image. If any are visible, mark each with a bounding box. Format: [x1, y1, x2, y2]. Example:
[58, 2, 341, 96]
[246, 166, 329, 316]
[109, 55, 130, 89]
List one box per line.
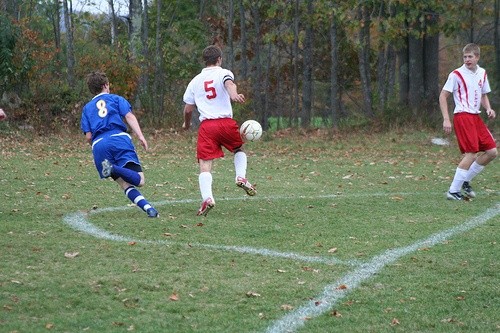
[80, 71, 160, 219]
[439, 43, 497, 202]
[181, 44, 258, 217]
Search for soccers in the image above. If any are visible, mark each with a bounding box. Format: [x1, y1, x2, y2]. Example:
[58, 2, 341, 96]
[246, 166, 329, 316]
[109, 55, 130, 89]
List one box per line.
[240, 119, 264, 143]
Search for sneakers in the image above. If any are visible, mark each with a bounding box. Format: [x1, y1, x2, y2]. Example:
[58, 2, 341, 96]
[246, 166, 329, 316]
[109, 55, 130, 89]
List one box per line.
[234, 177, 258, 196]
[102, 159, 111, 178]
[447, 190, 472, 202]
[145, 207, 158, 218]
[462, 183, 476, 198]
[196, 197, 216, 217]
